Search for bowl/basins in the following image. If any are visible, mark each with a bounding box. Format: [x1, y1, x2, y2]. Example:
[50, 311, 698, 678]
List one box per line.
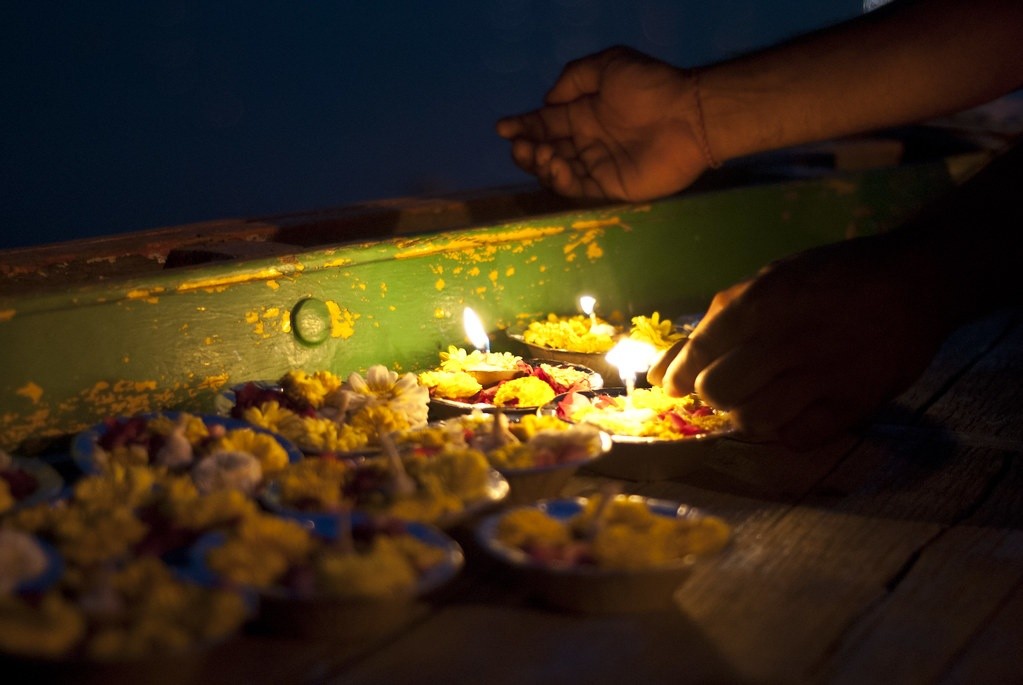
[0, 314, 740, 652]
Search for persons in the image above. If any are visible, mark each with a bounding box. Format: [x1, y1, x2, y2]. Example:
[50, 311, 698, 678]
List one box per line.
[496, 0, 1023, 450]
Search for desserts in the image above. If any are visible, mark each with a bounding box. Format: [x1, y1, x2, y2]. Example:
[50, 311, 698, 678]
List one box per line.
[0, 310, 747, 684]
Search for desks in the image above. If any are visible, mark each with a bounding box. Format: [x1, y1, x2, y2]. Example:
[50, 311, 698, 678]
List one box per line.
[329, 475, 1023, 685]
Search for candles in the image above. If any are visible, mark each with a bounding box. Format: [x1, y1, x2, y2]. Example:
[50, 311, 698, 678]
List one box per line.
[464, 307, 491, 354]
[580, 296, 599, 327]
[606, 336, 656, 396]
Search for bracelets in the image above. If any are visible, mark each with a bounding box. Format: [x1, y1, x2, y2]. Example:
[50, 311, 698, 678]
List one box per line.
[687, 71, 723, 170]
